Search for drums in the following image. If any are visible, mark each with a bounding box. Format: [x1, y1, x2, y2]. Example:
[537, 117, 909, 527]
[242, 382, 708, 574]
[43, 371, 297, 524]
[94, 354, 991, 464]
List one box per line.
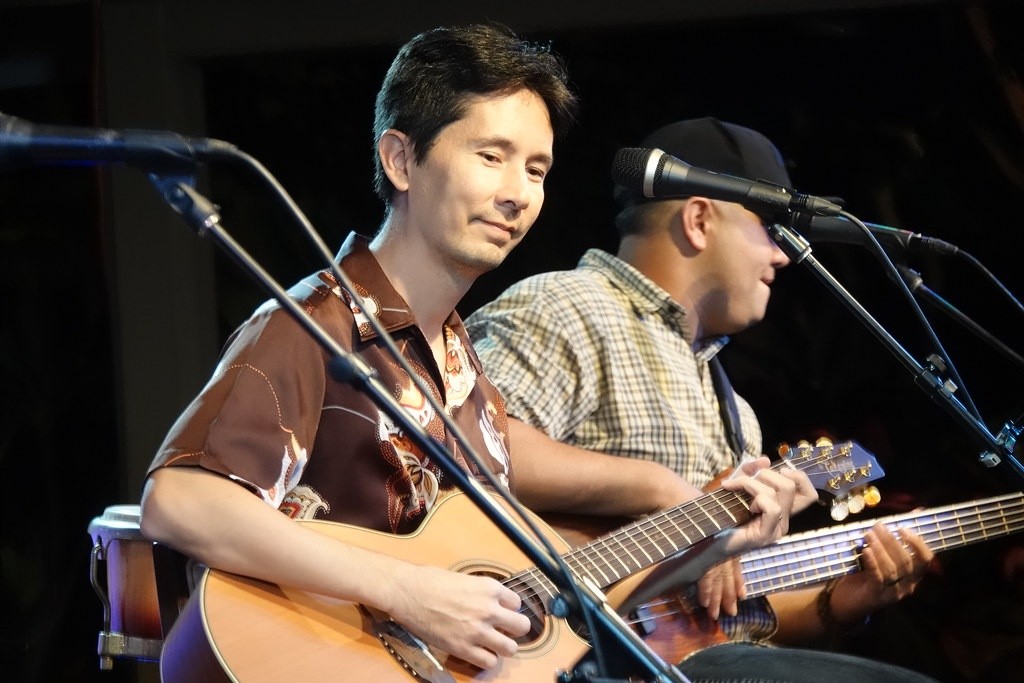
[89, 504, 159, 662]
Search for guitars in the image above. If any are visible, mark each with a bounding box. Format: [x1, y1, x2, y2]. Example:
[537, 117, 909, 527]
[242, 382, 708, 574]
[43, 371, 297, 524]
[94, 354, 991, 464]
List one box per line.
[160, 435, 1022, 683]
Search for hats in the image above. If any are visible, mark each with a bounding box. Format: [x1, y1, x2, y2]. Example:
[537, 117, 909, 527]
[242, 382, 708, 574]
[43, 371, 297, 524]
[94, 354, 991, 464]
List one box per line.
[612, 115, 849, 218]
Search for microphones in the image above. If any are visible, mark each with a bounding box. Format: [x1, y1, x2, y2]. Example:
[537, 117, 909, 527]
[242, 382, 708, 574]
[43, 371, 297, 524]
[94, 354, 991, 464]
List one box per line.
[0, 110, 237, 171]
[611, 147, 842, 220]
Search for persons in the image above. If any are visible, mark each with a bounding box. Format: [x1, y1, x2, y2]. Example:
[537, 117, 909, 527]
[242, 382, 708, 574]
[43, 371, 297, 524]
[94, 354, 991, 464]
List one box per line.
[465, 115, 947, 681]
[139, 22, 586, 674]
